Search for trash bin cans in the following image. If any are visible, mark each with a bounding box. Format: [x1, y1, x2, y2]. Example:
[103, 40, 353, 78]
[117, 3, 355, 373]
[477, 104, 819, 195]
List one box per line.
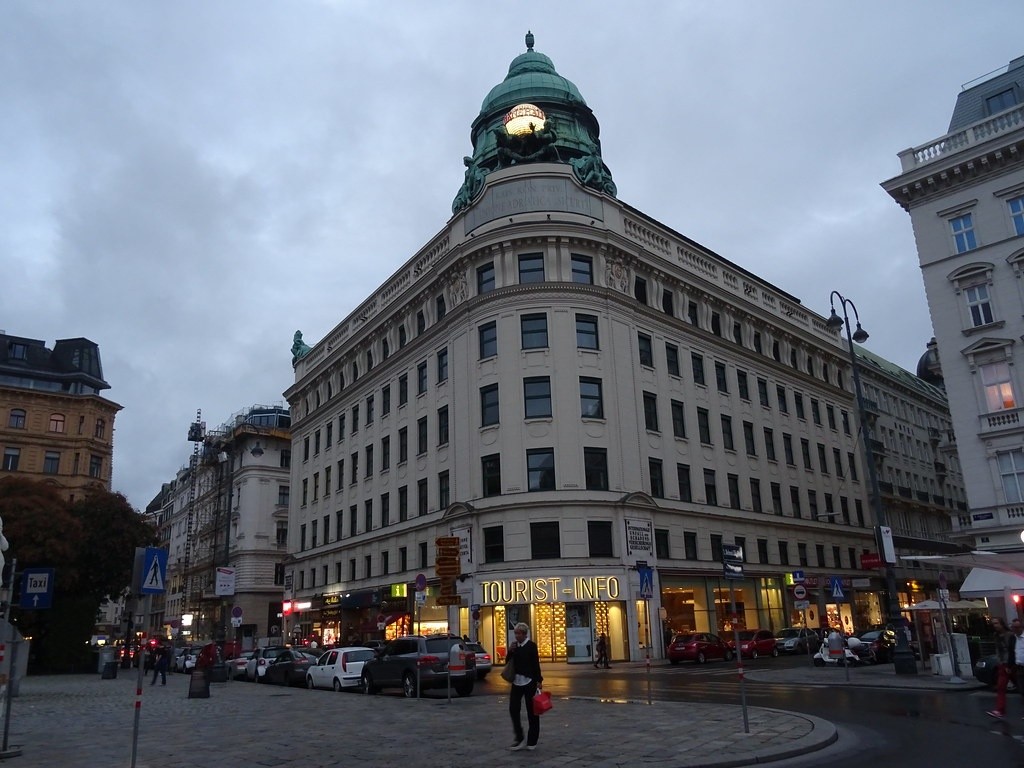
[829, 632, 845, 659]
[98, 649, 113, 673]
[449, 644, 466, 676]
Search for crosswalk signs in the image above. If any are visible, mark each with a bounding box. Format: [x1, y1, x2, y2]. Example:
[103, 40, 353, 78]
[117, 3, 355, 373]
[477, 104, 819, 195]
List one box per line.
[639, 567, 654, 599]
[141, 546, 170, 595]
[830, 577, 845, 601]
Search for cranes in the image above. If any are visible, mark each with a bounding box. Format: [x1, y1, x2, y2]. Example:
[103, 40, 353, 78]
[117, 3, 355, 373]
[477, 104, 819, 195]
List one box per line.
[181, 409, 206, 614]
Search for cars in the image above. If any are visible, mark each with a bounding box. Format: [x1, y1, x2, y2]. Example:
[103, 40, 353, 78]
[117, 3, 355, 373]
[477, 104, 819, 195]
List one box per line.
[361, 640, 386, 654]
[774, 627, 819, 655]
[727, 629, 776, 659]
[305, 646, 380, 692]
[859, 630, 916, 663]
[151, 645, 203, 674]
[464, 642, 492, 679]
[667, 632, 734, 665]
[224, 645, 334, 686]
[812, 627, 850, 651]
[974, 654, 1018, 692]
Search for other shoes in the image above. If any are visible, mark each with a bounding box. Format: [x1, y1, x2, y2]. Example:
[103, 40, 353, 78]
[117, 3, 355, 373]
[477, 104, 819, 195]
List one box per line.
[510, 736, 524, 750]
[985, 710, 1003, 720]
[594, 664, 598, 668]
[526, 740, 537, 750]
[604, 666, 611, 669]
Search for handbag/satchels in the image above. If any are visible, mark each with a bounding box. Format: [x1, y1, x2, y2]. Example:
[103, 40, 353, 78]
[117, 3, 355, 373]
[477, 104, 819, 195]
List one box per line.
[500, 642, 516, 682]
[531, 687, 553, 714]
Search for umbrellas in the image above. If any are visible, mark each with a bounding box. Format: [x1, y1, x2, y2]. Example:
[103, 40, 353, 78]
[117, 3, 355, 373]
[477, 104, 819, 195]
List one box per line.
[900, 599, 988, 634]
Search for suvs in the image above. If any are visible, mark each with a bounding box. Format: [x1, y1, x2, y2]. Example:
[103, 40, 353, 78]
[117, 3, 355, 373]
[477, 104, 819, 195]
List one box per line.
[361, 633, 477, 698]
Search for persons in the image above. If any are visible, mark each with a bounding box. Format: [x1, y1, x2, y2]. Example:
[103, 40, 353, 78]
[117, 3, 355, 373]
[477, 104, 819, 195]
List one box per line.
[724, 619, 734, 633]
[461, 156, 481, 205]
[147, 637, 175, 686]
[663, 625, 673, 646]
[594, 632, 611, 668]
[310, 636, 361, 649]
[582, 143, 604, 186]
[492, 118, 559, 147]
[985, 616, 1024, 720]
[463, 633, 473, 643]
[505, 622, 543, 750]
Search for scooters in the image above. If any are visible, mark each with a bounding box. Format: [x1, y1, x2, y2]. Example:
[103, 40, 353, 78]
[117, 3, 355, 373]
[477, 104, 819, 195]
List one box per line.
[814, 631, 861, 667]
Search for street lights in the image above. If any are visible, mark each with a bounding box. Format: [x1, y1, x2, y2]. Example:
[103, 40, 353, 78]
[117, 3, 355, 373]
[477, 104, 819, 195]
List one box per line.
[216, 422, 264, 646]
[826, 291, 918, 675]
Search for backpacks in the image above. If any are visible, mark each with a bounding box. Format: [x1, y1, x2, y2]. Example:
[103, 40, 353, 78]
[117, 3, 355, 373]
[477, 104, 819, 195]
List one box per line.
[596, 638, 604, 651]
